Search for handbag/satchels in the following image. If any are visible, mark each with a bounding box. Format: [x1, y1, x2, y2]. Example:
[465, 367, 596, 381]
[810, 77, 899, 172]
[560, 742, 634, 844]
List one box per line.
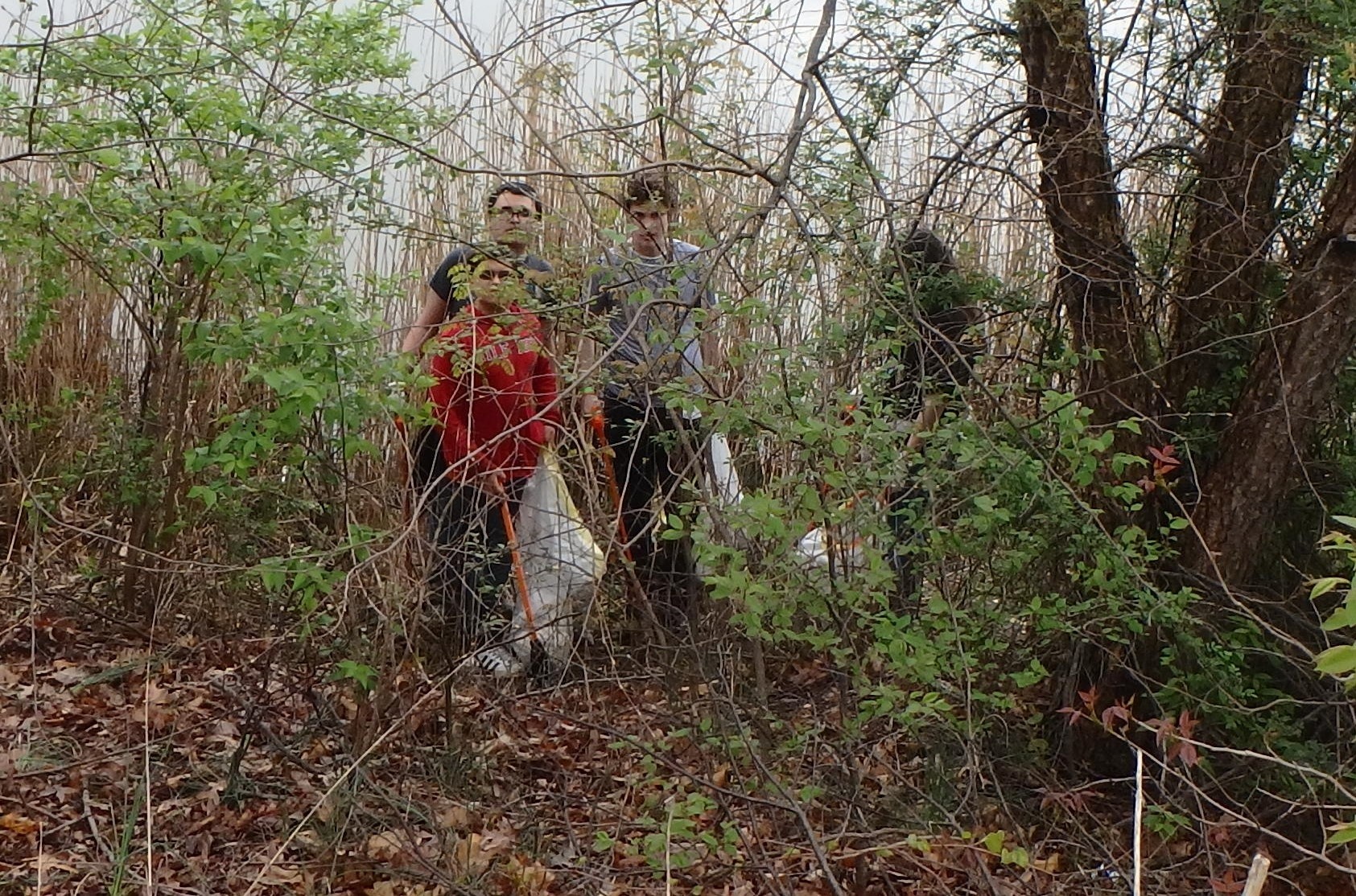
[500, 434, 606, 673]
[685, 427, 758, 590]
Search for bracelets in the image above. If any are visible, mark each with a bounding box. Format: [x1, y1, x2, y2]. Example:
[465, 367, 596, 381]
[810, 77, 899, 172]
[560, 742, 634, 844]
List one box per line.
[578, 386, 598, 396]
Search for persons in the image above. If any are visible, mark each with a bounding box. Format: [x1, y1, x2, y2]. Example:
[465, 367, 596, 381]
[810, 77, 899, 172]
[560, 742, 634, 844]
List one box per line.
[390, 181, 562, 618]
[577, 173, 729, 644]
[428, 242, 561, 681]
[861, 226, 974, 634]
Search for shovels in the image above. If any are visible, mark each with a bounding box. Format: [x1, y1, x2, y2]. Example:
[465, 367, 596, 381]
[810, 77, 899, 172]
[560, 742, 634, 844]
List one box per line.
[497, 481, 567, 689]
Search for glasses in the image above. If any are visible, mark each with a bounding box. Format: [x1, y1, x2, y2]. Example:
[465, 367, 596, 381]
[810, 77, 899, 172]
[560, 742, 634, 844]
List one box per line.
[487, 203, 545, 222]
[473, 268, 519, 284]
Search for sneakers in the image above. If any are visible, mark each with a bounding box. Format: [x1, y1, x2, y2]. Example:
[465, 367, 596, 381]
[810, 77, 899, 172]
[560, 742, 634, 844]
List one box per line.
[466, 647, 522, 680]
